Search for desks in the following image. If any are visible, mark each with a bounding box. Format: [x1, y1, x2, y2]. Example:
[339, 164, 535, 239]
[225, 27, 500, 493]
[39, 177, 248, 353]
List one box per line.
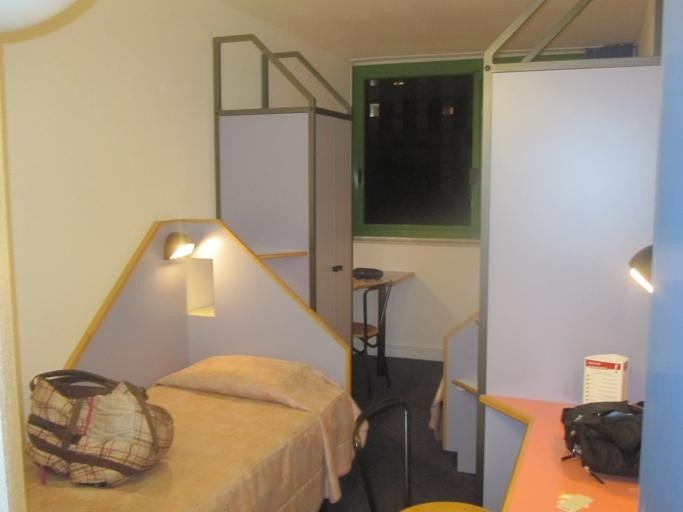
[352, 268, 414, 377]
[479, 395, 642, 512]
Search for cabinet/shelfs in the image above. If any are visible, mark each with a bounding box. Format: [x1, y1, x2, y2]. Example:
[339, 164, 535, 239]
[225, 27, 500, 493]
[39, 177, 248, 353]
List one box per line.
[213, 33, 352, 345]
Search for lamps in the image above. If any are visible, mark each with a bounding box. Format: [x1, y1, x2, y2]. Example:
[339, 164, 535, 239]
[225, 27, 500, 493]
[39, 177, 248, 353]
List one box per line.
[628, 244, 652, 293]
[164, 233, 195, 261]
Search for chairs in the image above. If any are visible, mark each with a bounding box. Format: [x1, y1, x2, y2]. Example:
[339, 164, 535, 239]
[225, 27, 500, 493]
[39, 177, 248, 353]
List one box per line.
[353, 397, 494, 512]
[353, 282, 390, 397]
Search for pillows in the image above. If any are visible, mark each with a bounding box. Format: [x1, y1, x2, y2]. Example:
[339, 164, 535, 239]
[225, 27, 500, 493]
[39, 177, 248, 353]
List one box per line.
[160, 354, 343, 413]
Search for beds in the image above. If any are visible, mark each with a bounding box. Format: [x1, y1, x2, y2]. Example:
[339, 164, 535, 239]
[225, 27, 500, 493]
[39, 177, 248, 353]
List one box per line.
[16, 218, 351, 512]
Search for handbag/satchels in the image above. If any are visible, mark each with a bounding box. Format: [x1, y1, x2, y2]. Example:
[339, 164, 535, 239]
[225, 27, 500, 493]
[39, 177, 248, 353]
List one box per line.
[25, 370, 172, 487]
[561, 401, 642, 478]
